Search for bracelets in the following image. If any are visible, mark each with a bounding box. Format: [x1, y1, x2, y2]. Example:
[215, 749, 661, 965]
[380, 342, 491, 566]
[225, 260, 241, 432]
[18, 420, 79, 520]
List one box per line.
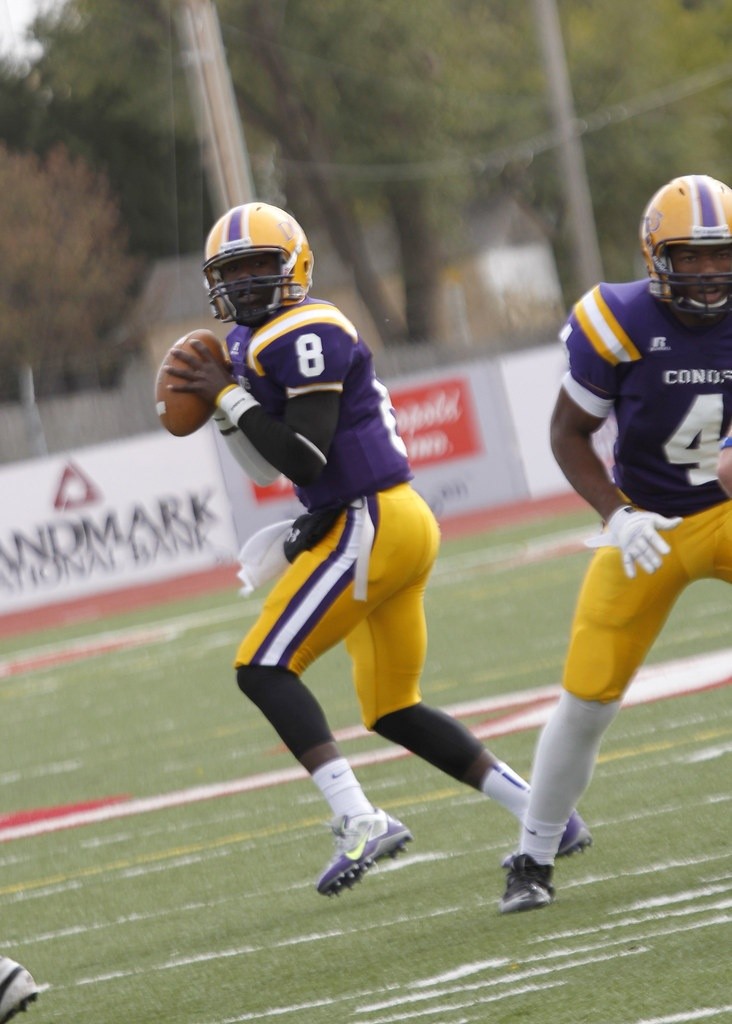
[216, 383, 239, 408]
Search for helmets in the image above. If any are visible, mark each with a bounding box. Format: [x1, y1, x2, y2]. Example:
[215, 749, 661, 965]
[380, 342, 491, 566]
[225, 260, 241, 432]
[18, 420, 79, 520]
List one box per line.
[204, 202, 313, 323]
[640, 175, 732, 315]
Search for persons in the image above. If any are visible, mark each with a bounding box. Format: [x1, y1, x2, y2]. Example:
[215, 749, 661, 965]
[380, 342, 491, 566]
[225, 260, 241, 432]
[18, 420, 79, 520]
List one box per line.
[497, 174, 730, 914]
[162, 202, 593, 898]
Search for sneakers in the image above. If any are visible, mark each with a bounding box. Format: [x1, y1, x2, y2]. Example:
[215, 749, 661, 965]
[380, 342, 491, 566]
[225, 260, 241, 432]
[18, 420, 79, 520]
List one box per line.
[0, 957, 39, 1024]
[318, 808, 413, 897]
[500, 854, 555, 915]
[555, 812, 592, 859]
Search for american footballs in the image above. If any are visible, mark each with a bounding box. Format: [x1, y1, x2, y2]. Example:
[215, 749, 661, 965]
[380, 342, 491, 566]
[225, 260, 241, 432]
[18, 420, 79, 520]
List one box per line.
[152, 326, 225, 438]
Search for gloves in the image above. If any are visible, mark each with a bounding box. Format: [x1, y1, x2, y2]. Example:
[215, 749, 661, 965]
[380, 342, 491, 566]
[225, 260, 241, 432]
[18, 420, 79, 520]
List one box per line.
[605, 505, 683, 578]
